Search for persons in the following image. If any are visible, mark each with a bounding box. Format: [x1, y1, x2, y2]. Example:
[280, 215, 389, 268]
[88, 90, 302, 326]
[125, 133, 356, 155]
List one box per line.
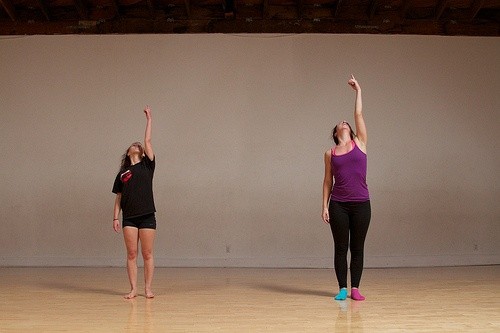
[111, 104, 157, 299]
[321, 74, 372, 301]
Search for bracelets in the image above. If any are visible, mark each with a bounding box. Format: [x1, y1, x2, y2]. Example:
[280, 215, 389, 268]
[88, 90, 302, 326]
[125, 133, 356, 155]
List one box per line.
[113, 219, 119, 221]
